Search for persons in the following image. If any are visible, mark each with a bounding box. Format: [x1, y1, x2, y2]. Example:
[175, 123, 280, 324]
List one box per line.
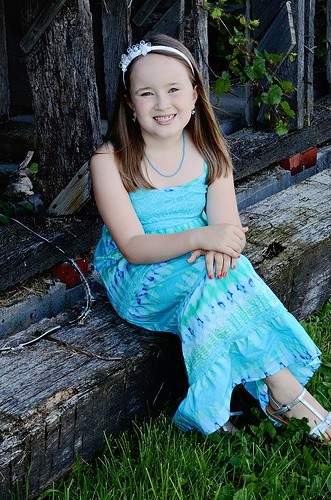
[90, 35, 330, 446]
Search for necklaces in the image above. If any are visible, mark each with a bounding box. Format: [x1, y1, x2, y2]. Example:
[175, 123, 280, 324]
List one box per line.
[140, 130, 187, 179]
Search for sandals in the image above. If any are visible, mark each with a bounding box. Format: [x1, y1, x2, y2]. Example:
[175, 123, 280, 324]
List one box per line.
[221, 420, 239, 436]
[266, 387, 331, 445]
